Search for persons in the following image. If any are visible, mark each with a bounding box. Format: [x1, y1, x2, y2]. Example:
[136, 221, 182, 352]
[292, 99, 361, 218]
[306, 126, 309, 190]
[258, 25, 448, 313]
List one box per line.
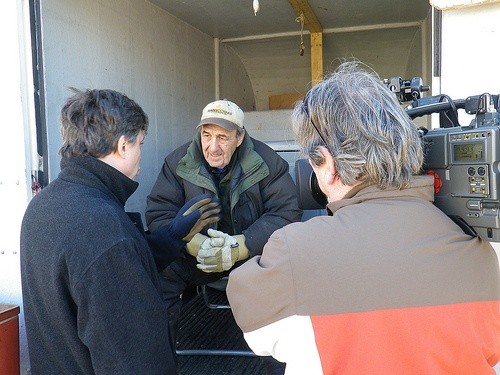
[20, 89, 179, 375]
[226, 57, 500, 375]
[146, 100, 303, 273]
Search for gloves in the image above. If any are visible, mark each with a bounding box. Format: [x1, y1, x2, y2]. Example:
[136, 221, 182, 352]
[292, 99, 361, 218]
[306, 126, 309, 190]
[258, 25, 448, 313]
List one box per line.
[196, 227, 240, 273]
[164, 193, 221, 240]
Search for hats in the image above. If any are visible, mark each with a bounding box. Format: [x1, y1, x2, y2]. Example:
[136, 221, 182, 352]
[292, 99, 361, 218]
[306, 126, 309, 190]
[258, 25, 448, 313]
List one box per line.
[196, 98, 245, 132]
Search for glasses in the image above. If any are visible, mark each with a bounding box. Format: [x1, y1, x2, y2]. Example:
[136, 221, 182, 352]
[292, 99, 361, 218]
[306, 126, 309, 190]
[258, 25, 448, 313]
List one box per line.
[302, 86, 331, 156]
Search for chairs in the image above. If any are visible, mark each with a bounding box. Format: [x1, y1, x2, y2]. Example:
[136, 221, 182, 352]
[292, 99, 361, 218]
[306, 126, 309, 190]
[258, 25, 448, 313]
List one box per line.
[175, 276, 260, 357]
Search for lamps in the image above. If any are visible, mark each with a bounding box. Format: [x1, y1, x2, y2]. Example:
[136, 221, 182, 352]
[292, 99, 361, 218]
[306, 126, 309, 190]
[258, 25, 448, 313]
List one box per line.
[252, 0, 259, 17]
